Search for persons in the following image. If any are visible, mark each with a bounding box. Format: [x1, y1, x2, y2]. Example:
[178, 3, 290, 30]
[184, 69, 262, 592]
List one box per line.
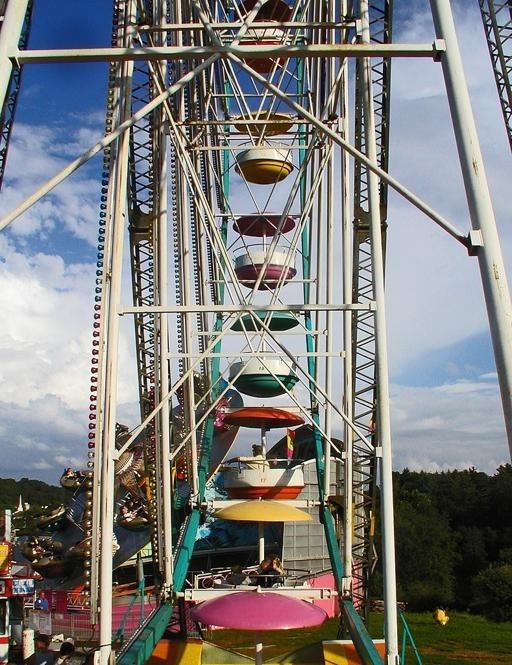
[224, 557, 287, 587]
[224, 445, 270, 470]
[120, 500, 150, 522]
[270, 456, 283, 469]
[34, 590, 49, 610]
[30, 537, 46, 559]
[54, 641, 76, 665]
[64, 468, 86, 483]
[23, 635, 57, 665]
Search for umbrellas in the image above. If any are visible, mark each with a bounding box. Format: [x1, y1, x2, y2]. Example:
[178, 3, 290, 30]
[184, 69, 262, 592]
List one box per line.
[230, 311, 299, 360]
[234, 212, 295, 253]
[222, 407, 306, 457]
[233, 0, 293, 23]
[234, 111, 292, 144]
[212, 497, 312, 564]
[189, 589, 328, 665]
